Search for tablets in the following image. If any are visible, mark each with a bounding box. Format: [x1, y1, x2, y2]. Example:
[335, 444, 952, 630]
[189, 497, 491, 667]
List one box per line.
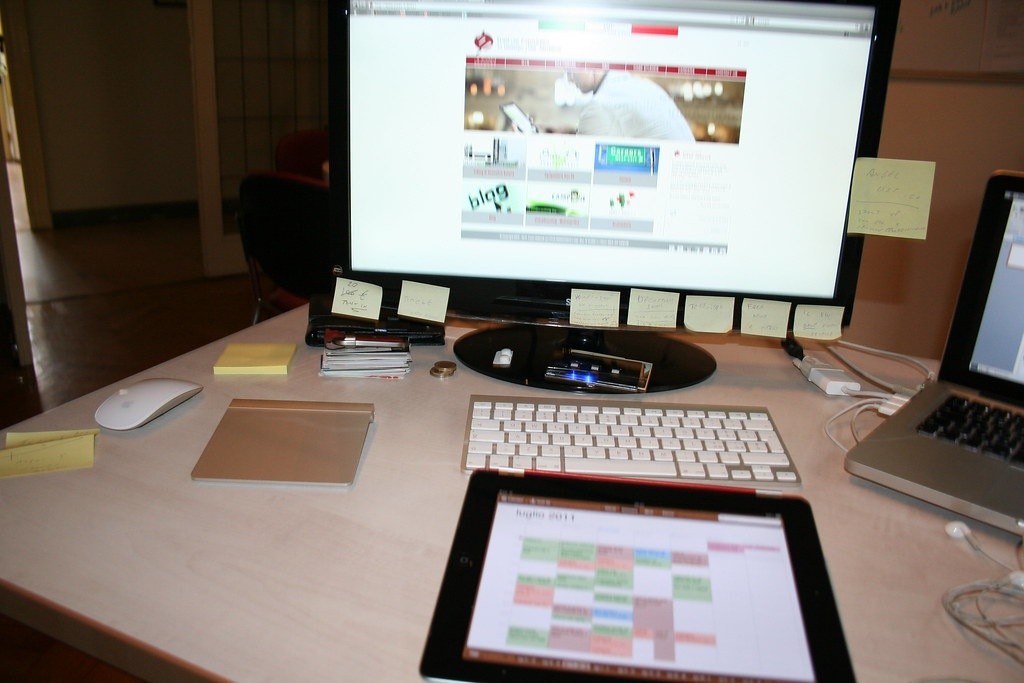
[420, 467, 857, 683]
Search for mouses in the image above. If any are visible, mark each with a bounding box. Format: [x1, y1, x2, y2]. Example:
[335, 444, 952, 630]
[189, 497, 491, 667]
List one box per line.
[94, 377, 204, 431]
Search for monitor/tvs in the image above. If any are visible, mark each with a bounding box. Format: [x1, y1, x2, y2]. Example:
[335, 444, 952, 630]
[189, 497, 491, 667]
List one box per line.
[329, 0, 902, 328]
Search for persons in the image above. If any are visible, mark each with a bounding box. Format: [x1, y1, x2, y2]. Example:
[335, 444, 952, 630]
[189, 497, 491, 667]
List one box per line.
[567, 68, 697, 143]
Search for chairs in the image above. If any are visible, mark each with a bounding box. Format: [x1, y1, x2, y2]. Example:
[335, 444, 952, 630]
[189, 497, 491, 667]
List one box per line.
[235, 165, 349, 325]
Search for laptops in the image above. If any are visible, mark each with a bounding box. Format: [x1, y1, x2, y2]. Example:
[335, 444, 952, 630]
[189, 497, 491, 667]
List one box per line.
[843, 169, 1024, 539]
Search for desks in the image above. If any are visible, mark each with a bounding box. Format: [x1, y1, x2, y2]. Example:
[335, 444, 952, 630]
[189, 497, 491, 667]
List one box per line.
[1, 293, 1023, 681]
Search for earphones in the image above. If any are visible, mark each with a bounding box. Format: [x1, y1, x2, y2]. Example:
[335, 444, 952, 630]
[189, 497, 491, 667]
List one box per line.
[992, 570, 1024, 589]
[944, 520, 981, 551]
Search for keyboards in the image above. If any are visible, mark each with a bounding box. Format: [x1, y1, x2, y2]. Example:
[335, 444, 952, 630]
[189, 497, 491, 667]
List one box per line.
[460, 393, 806, 493]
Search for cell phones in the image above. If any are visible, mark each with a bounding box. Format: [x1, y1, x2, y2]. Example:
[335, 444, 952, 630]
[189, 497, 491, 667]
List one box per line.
[499, 102, 539, 134]
